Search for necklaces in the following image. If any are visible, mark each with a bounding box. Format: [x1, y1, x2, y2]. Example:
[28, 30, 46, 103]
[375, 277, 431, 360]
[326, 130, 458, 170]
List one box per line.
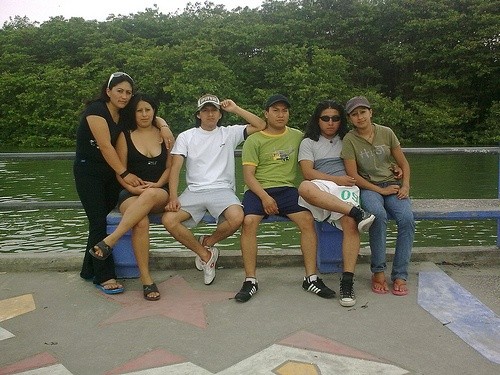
[322, 134, 333, 143]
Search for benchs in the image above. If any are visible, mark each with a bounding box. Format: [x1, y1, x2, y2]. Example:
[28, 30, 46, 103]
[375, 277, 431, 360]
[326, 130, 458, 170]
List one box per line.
[106, 199, 500, 281]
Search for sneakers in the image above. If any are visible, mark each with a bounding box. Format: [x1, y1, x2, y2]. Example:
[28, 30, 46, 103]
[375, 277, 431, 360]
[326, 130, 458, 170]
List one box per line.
[200, 243, 220, 285]
[302, 274, 336, 299]
[228, 277, 259, 303]
[351, 206, 376, 232]
[195, 235, 214, 271]
[339, 276, 356, 307]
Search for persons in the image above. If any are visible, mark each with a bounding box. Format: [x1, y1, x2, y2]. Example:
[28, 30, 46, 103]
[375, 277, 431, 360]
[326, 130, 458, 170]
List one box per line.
[234, 95, 416, 308]
[160, 93, 267, 284]
[73, 72, 176, 301]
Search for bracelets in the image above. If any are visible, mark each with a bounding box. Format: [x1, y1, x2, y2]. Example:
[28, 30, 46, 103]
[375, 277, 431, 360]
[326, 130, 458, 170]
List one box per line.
[160, 125, 169, 129]
[120, 170, 129, 178]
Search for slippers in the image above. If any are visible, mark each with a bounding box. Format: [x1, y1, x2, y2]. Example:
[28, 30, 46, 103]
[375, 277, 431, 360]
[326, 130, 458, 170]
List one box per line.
[371, 273, 389, 294]
[89, 241, 114, 261]
[143, 282, 160, 301]
[391, 280, 408, 296]
[96, 281, 126, 295]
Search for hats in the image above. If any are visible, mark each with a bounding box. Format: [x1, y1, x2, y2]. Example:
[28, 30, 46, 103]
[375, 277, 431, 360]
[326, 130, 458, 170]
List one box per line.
[346, 96, 371, 114]
[266, 94, 291, 109]
[197, 94, 221, 111]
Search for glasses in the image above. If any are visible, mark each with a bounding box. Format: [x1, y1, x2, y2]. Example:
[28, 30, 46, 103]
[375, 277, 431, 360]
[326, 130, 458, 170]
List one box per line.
[319, 115, 341, 122]
[107, 72, 134, 88]
